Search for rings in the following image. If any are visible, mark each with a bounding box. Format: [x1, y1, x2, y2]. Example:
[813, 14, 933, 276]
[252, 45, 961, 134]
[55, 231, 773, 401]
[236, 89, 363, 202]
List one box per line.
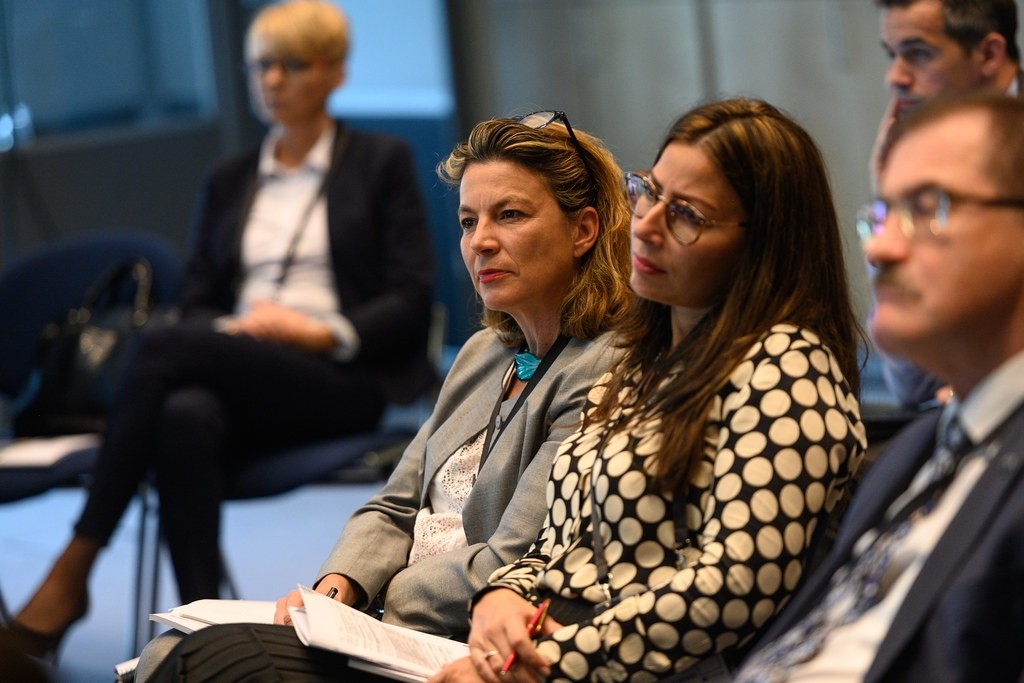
[483, 651, 499, 659]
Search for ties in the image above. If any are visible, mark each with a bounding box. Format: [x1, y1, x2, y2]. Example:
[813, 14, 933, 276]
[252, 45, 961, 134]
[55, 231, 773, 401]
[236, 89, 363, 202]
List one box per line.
[732, 404, 973, 683]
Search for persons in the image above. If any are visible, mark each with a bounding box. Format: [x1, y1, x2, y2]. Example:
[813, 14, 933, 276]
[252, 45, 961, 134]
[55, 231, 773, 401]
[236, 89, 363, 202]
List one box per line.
[144, 97, 868, 683]
[714, 93, 1024, 682]
[869, 0, 1024, 406]
[0, 1, 435, 660]
[133, 110, 638, 683]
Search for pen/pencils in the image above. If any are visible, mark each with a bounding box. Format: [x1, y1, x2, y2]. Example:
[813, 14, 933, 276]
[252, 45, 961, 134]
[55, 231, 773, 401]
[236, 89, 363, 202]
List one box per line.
[326, 586, 339, 600]
[498, 598, 552, 676]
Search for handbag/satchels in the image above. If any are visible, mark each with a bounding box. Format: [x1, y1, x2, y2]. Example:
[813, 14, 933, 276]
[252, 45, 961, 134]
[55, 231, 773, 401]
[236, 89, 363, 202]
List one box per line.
[11, 253, 182, 437]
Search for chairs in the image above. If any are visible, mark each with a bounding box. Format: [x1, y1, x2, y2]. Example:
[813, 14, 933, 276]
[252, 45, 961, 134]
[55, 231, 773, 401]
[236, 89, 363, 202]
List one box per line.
[0, 225, 446, 664]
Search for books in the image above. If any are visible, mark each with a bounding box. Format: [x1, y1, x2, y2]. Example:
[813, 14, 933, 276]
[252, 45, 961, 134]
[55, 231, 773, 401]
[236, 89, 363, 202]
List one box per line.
[148, 596, 277, 635]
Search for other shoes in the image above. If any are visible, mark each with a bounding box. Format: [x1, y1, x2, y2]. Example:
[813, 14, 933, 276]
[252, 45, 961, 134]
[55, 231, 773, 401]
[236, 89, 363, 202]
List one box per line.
[0, 597, 90, 681]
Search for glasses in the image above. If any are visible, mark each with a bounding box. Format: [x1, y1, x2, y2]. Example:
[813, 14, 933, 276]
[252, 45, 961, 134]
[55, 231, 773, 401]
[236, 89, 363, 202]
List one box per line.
[249, 56, 314, 72]
[854, 180, 1024, 242]
[624, 169, 746, 249]
[512, 110, 598, 194]
